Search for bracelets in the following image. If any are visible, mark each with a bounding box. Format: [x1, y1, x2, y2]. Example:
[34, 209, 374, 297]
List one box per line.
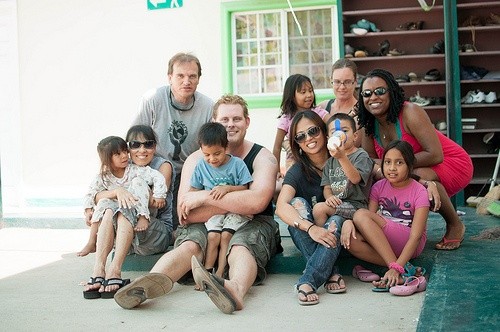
[306, 223, 315, 232]
[424, 180, 428, 188]
[294, 215, 304, 229]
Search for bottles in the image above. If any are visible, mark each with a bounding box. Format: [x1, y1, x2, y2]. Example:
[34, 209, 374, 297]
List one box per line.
[327, 119, 348, 151]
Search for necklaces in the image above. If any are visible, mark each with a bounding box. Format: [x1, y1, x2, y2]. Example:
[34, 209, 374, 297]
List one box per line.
[383, 134, 386, 138]
[169, 88, 195, 111]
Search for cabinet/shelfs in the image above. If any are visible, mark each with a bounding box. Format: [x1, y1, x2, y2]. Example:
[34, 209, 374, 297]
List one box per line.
[337, 0, 500, 206]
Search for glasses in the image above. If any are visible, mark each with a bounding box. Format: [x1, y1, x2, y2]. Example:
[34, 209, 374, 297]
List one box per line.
[294, 126, 321, 143]
[127, 139, 154, 150]
[361, 87, 388, 97]
[331, 79, 357, 87]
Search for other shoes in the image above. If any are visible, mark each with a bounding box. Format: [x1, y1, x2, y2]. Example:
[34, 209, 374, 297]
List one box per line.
[389, 276, 426, 296]
[353, 268, 380, 281]
[341, 17, 500, 153]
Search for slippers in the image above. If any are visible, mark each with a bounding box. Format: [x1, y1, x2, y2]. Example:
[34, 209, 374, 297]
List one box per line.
[100, 278, 131, 298]
[371, 279, 390, 292]
[402, 262, 427, 277]
[434, 236, 465, 250]
[191, 255, 236, 313]
[84, 276, 105, 298]
[323, 276, 347, 294]
[298, 288, 319, 304]
[114, 273, 173, 309]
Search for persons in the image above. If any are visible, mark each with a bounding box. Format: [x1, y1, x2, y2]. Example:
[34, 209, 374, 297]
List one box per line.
[76, 53, 374, 314]
[340, 140, 431, 293]
[352, 69, 473, 251]
